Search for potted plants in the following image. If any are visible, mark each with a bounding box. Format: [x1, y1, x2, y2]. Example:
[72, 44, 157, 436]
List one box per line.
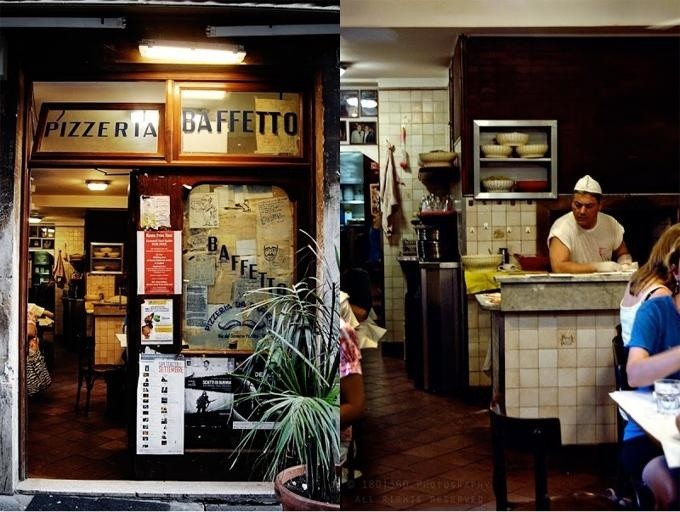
[226, 229, 341, 511]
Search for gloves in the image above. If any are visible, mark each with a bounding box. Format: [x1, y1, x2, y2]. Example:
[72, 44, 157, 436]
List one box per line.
[617, 253, 633, 271]
[593, 261, 623, 272]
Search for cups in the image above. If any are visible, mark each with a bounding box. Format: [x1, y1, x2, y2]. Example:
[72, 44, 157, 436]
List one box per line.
[340, 187, 364, 201]
[652, 379, 679, 417]
[35, 267, 49, 273]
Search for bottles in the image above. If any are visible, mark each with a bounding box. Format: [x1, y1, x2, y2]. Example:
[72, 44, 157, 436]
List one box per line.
[418, 192, 455, 214]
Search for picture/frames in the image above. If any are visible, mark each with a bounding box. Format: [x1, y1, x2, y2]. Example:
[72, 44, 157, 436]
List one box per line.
[339, 88, 378, 145]
[28, 225, 55, 249]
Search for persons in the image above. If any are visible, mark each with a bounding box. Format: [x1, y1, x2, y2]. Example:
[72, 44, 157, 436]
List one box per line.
[349, 124, 364, 143]
[619, 220, 680, 347]
[362, 124, 376, 144]
[622, 244, 680, 481]
[340, 321, 366, 464]
[544, 173, 633, 274]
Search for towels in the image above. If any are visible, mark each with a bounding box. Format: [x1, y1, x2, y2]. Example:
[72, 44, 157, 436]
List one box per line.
[380, 140, 398, 237]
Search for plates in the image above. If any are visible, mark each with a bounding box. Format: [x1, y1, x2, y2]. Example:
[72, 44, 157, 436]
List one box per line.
[345, 96, 378, 117]
[402, 225, 460, 261]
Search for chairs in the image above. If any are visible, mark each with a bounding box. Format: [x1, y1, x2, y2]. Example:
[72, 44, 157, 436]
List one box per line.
[75, 334, 120, 418]
[489, 323, 680, 511]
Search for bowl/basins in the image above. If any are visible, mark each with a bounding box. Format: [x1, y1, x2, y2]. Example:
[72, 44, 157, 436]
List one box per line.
[496, 132, 529, 146]
[94, 252, 105, 256]
[106, 266, 121, 271]
[480, 144, 514, 158]
[93, 266, 106, 270]
[107, 252, 121, 258]
[419, 151, 458, 167]
[461, 254, 503, 272]
[100, 248, 112, 252]
[70, 255, 82, 261]
[516, 180, 548, 193]
[483, 179, 513, 192]
[519, 256, 552, 272]
[515, 144, 549, 157]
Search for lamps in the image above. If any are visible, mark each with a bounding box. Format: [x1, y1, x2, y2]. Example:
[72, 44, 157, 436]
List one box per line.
[139, 32, 247, 67]
[86, 180, 111, 191]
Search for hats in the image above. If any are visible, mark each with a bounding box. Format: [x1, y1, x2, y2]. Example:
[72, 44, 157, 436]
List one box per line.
[574, 175, 603, 195]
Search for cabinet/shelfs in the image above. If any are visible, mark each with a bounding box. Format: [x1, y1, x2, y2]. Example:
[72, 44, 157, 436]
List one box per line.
[341, 184, 365, 226]
[29, 251, 54, 288]
[90, 242, 123, 275]
[474, 119, 557, 200]
